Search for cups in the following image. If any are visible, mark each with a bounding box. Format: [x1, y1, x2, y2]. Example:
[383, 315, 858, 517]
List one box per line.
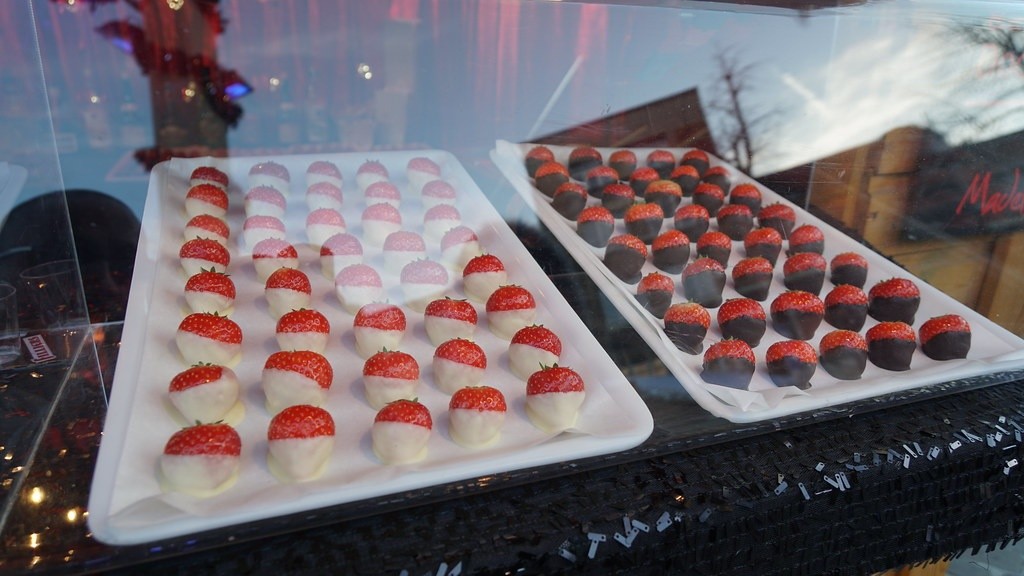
[0, 283, 22, 364]
[21, 258, 81, 337]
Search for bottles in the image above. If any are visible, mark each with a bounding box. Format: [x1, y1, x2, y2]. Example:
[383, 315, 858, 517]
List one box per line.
[79, 66, 148, 147]
[233, 68, 329, 147]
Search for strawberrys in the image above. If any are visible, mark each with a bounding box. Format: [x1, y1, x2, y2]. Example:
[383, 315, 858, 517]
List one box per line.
[526, 146, 972, 387]
[158, 158, 584, 503]
